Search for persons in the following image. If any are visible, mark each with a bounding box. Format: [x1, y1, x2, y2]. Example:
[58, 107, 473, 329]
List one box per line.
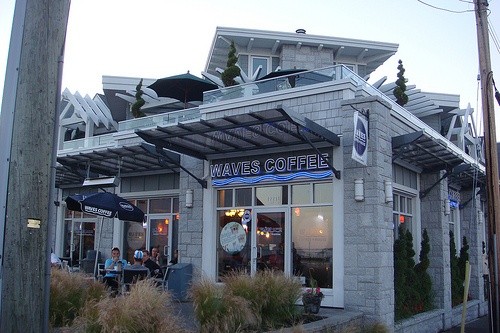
[51, 248, 62, 268]
[293, 248, 301, 271]
[231, 244, 284, 275]
[104, 246, 178, 297]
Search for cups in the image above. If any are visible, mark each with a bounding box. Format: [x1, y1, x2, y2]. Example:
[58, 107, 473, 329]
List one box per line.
[113, 266, 118, 270]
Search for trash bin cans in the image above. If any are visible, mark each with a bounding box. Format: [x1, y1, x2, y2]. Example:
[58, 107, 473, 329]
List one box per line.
[169, 263, 194, 302]
[82, 250, 102, 280]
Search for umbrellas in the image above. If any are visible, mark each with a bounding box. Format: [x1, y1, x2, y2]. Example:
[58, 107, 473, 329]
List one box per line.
[147, 70, 220, 109]
[254, 67, 333, 94]
[220, 212, 280, 244]
[66, 191, 147, 278]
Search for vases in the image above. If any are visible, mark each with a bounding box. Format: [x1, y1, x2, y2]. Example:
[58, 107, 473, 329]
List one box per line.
[302, 297, 322, 314]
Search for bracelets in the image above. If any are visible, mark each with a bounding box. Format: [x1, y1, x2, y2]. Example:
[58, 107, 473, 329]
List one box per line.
[156, 260, 159, 261]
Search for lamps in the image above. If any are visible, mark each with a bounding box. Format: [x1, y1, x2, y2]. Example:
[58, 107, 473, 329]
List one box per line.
[186, 188, 194, 208]
[354, 178, 365, 202]
[384, 180, 393, 202]
[478, 211, 482, 223]
[445, 199, 450, 215]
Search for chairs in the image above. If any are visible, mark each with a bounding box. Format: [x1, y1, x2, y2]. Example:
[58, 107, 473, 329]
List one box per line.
[98, 263, 171, 290]
[79, 250, 97, 273]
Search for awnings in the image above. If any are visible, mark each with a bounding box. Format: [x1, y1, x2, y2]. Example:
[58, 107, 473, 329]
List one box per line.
[54, 105, 341, 187]
[391, 131, 486, 209]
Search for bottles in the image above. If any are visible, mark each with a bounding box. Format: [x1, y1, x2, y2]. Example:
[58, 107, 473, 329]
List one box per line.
[118, 260, 125, 271]
[127, 262, 132, 267]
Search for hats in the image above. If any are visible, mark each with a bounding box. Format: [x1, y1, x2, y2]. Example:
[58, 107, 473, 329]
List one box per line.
[134, 251, 143, 258]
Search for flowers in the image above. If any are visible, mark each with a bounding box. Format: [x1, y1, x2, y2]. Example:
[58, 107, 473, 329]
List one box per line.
[302, 278, 324, 304]
